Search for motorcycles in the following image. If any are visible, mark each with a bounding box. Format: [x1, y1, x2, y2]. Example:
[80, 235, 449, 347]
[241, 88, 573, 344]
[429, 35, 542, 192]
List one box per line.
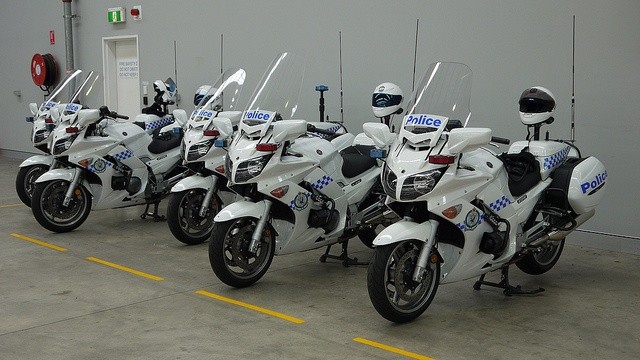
[167, 31, 347, 244]
[366, 16, 608, 323]
[209, 20, 418, 287]
[16, 40, 178, 208]
[32, 34, 223, 232]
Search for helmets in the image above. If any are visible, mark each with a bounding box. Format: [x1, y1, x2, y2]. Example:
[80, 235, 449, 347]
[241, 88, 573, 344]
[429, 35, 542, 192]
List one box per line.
[194, 85, 220, 109]
[519, 87, 556, 124]
[372, 83, 403, 117]
[153, 77, 177, 101]
